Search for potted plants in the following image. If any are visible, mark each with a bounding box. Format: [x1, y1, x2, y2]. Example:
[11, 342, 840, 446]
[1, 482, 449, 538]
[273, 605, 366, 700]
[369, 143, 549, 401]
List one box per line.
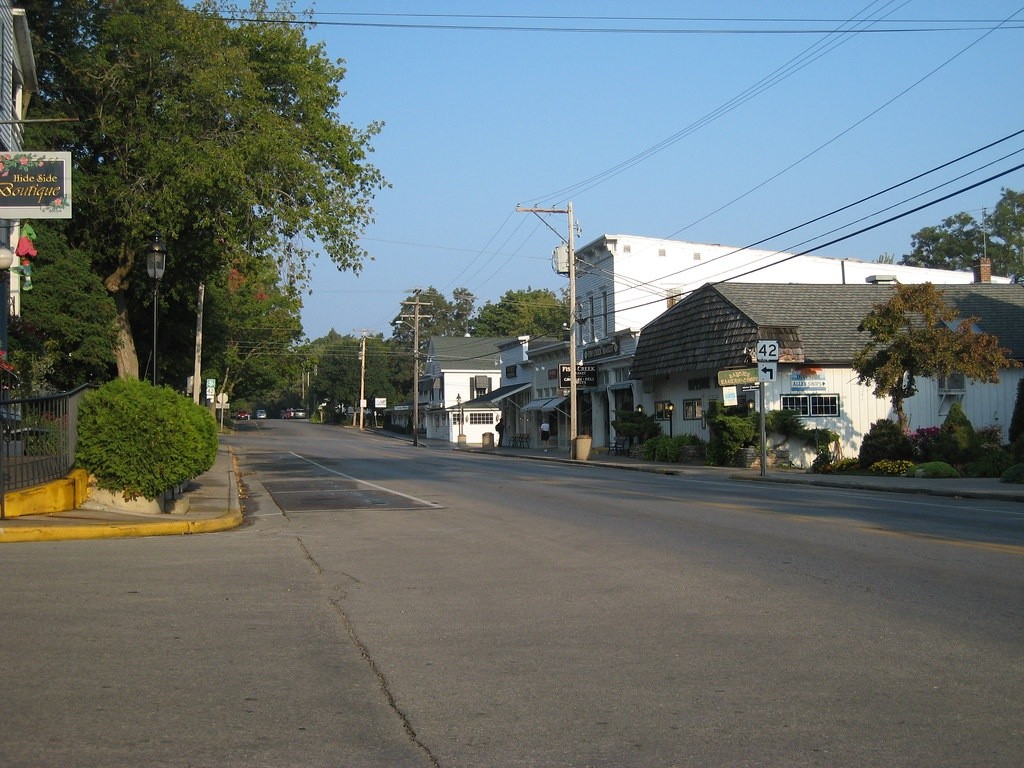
[611, 408, 705, 463]
[715, 415, 758, 468]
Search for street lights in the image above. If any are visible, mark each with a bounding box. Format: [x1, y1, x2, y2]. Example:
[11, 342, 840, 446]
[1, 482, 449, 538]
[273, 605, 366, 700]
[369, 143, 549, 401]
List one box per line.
[394, 320, 418, 447]
[146, 233, 166, 386]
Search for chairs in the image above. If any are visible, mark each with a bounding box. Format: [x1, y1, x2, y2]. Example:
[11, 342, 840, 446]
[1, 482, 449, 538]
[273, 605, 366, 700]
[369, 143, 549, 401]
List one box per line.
[509, 432, 530, 449]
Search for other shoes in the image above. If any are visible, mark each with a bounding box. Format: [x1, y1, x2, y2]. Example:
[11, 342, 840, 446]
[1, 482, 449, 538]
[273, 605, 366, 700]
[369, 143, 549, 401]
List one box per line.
[544, 449, 547, 452]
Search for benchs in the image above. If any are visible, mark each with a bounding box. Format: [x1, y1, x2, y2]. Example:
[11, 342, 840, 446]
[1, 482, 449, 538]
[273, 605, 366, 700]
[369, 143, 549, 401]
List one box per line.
[608, 436, 631, 457]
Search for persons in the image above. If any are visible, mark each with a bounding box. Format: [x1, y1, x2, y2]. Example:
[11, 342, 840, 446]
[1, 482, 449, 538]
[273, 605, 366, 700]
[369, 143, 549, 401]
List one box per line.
[538, 418, 550, 453]
[495, 418, 504, 447]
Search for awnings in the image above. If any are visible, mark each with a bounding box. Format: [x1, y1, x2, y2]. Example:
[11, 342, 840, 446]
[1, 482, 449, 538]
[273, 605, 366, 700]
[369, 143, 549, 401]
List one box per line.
[465, 383, 532, 415]
[521, 396, 570, 419]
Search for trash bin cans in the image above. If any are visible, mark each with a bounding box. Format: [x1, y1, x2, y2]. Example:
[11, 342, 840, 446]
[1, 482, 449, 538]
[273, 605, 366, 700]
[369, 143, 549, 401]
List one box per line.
[458, 435, 466, 447]
[482, 433, 494, 451]
[575, 435, 593, 460]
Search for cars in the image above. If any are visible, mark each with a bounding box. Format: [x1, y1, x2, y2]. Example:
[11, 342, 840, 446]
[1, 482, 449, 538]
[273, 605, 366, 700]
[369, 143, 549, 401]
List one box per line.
[256, 410, 267, 419]
[236, 410, 248, 420]
[294, 409, 306, 419]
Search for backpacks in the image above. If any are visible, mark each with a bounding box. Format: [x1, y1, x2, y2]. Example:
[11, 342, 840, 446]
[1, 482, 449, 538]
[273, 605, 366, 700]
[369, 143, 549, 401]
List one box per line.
[496, 423, 501, 431]
